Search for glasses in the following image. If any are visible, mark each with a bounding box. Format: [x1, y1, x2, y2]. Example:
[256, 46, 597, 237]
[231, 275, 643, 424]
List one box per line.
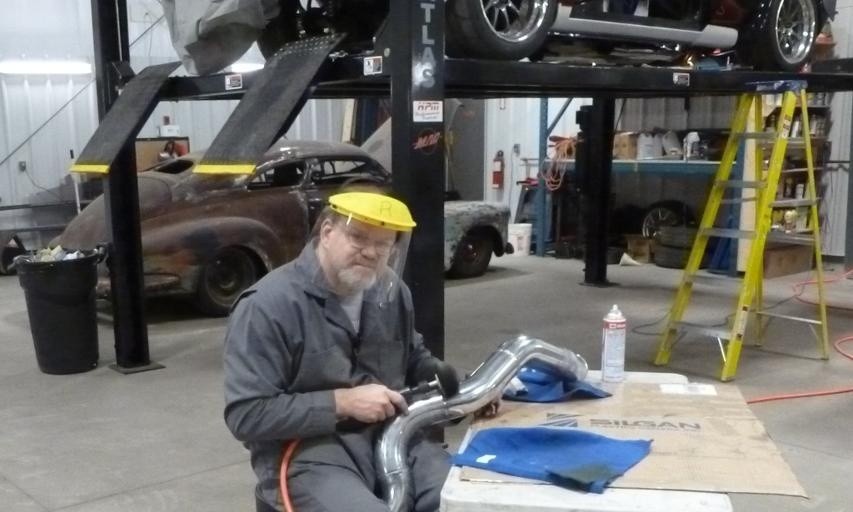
[334, 217, 398, 256]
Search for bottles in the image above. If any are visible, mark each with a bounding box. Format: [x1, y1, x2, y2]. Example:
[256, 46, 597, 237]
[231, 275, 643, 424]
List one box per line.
[768, 113, 828, 137]
[601, 303, 626, 383]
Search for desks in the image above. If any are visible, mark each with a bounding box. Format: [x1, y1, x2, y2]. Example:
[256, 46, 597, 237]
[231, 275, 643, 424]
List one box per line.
[544, 155, 738, 277]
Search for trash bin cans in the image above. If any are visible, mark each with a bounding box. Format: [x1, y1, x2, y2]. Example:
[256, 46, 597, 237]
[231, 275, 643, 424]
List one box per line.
[13, 248, 98, 375]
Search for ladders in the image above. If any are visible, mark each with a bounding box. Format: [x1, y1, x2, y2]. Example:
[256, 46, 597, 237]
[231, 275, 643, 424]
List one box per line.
[655, 82, 836, 381]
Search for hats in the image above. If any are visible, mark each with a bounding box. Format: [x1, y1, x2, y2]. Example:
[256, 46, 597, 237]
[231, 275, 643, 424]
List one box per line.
[327, 190, 418, 233]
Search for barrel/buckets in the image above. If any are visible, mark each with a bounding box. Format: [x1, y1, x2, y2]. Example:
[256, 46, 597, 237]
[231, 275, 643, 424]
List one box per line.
[508, 223, 533, 257]
[635, 132, 683, 160]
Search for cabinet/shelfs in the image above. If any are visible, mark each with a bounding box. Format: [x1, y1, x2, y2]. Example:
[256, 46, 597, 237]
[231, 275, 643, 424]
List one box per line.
[759, 93, 830, 234]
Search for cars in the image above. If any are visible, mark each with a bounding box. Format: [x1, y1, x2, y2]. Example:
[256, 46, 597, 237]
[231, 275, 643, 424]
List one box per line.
[256, 1, 835, 72]
[48, 99, 513, 317]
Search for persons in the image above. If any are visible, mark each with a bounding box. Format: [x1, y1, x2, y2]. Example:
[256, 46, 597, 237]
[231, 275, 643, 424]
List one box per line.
[222, 182, 504, 512]
[157, 140, 178, 162]
